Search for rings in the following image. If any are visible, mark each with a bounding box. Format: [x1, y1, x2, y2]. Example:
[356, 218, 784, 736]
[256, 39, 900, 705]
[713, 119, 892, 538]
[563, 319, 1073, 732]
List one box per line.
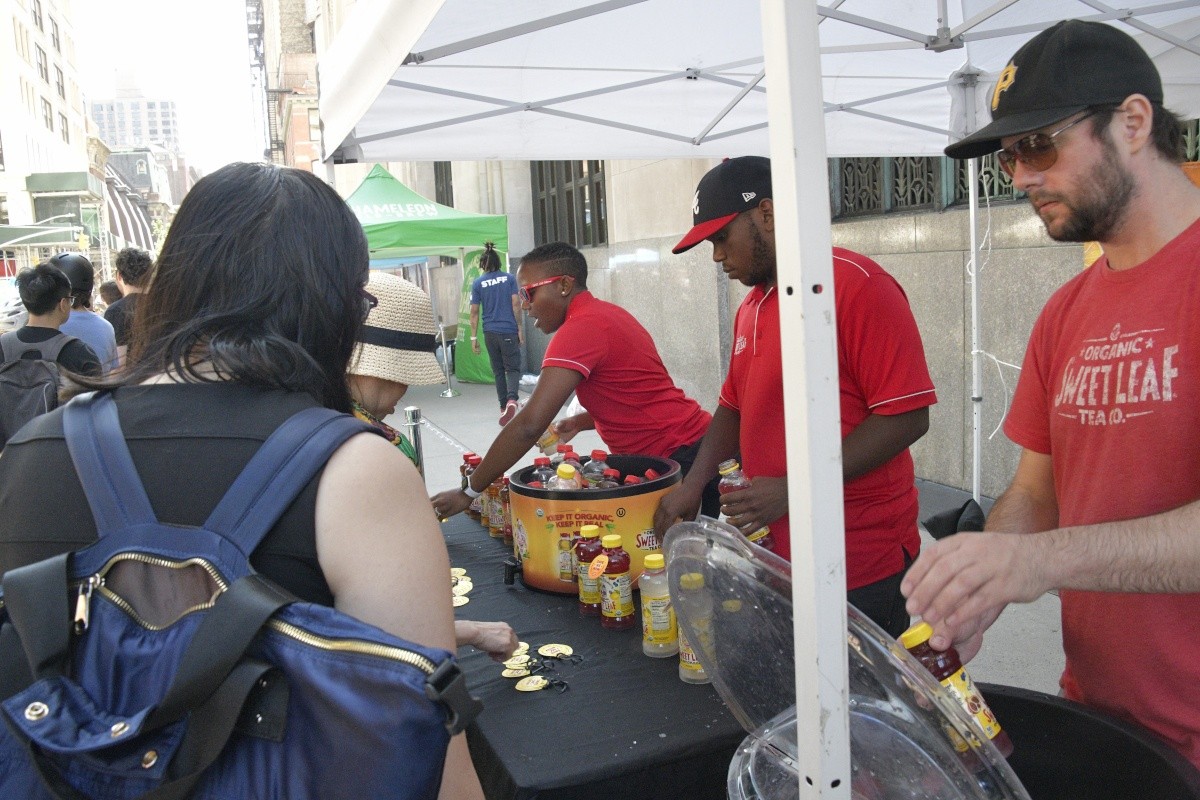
[434, 507, 441, 516]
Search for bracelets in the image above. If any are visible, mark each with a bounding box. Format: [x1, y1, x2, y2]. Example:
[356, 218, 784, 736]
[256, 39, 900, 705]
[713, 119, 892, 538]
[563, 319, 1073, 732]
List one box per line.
[470, 337, 477, 340]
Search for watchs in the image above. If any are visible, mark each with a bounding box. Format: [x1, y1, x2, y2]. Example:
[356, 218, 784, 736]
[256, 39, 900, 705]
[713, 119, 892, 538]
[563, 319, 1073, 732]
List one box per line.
[461, 474, 482, 499]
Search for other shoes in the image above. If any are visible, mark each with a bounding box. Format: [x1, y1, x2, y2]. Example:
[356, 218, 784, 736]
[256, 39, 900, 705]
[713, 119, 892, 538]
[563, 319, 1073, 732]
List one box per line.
[498, 399, 518, 426]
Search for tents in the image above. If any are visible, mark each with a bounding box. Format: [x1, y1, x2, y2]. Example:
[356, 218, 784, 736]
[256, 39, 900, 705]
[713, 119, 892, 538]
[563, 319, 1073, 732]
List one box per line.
[341, 162, 511, 385]
[313, 0, 1200, 515]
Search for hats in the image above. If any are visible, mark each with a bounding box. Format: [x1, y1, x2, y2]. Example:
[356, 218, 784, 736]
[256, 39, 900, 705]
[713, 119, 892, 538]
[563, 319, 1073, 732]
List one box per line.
[941, 19, 1165, 159]
[671, 156, 772, 255]
[346, 269, 448, 387]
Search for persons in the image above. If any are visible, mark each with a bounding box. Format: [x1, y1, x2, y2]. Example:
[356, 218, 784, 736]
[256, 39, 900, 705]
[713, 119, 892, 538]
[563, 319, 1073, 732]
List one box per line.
[428, 240, 742, 523]
[0, 247, 158, 456]
[899, 20, 1200, 772]
[0, 162, 493, 800]
[345, 270, 520, 664]
[470, 243, 525, 426]
[652, 155, 937, 645]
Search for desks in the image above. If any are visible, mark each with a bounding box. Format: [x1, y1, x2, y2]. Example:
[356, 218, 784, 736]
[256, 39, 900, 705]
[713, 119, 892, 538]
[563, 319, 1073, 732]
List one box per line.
[439, 509, 755, 800]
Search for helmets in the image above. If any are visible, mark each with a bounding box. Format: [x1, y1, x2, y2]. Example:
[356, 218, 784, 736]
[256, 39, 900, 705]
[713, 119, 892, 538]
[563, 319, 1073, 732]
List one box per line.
[47, 252, 94, 290]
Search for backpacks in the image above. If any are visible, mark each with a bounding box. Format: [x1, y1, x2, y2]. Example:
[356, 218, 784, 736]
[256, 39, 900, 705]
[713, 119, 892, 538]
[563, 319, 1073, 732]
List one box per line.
[0, 391, 481, 800]
[0, 332, 79, 440]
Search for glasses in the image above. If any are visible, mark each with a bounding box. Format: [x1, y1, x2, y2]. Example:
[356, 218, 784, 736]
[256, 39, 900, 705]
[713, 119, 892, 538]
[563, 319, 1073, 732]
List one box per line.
[997, 103, 1121, 179]
[518, 275, 575, 304]
[64, 296, 76, 306]
[358, 287, 379, 323]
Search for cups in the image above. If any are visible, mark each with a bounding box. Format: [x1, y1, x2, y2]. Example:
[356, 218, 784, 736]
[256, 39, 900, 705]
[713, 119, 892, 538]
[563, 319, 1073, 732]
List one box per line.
[430, 494, 448, 523]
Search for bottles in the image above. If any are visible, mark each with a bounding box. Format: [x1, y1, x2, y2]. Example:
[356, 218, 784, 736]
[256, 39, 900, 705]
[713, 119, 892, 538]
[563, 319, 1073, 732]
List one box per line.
[481, 486, 489, 527]
[527, 481, 540, 488]
[677, 573, 718, 685]
[639, 554, 678, 658]
[520, 398, 565, 456]
[600, 469, 620, 489]
[464, 456, 482, 520]
[500, 477, 514, 546]
[562, 452, 583, 489]
[551, 444, 573, 475]
[576, 525, 603, 616]
[641, 468, 660, 483]
[531, 457, 556, 490]
[559, 531, 580, 582]
[459, 452, 476, 514]
[900, 622, 1014, 774]
[599, 534, 637, 630]
[718, 458, 776, 550]
[623, 475, 641, 487]
[582, 450, 610, 489]
[489, 472, 505, 537]
[547, 463, 579, 490]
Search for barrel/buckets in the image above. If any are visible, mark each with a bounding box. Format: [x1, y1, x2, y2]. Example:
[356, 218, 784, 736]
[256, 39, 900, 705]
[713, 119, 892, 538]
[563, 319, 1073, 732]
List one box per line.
[504, 454, 683, 599]
